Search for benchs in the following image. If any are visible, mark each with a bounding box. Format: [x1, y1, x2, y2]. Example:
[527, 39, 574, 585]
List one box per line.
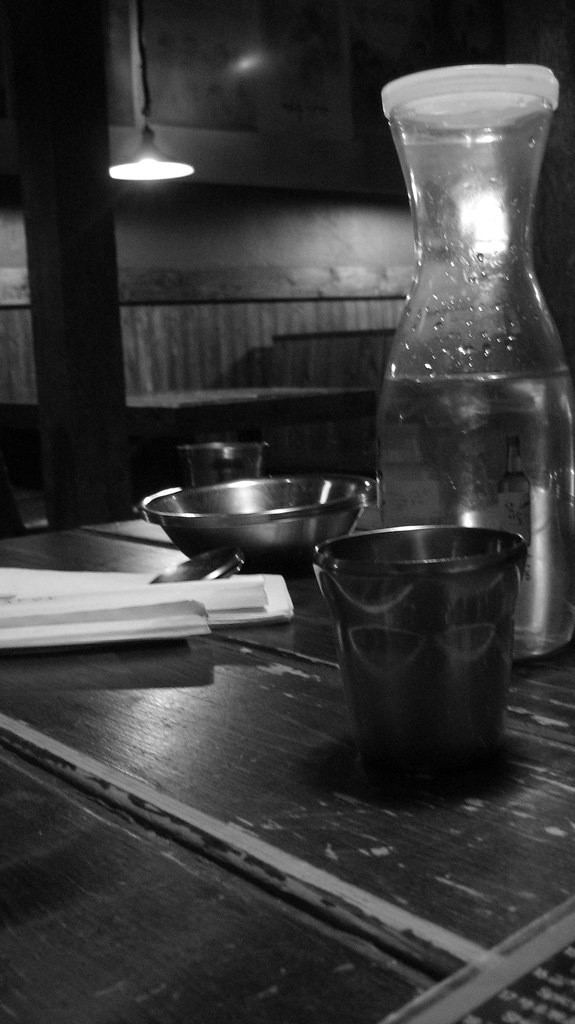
[271, 329, 399, 481]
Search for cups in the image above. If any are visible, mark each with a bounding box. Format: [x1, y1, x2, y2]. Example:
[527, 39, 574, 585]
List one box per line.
[313, 526, 528, 793]
[178, 438, 268, 493]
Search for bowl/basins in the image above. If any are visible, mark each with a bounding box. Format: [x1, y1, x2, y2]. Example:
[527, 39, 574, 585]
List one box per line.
[141, 473, 377, 574]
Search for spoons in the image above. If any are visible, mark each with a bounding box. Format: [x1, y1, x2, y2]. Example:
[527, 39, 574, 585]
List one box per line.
[0, 545, 246, 607]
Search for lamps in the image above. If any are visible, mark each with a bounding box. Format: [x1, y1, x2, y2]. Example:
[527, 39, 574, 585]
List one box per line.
[106, 0, 195, 180]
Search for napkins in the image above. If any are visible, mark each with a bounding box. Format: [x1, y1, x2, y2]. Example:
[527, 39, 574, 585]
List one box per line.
[0, 568, 294, 649]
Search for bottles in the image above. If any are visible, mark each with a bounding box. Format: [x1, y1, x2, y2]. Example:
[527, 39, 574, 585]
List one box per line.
[366, 62, 574, 663]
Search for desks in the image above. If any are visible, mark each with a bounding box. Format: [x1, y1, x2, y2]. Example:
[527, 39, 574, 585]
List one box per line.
[0, 518, 575, 1024]
[1, 387, 381, 522]
